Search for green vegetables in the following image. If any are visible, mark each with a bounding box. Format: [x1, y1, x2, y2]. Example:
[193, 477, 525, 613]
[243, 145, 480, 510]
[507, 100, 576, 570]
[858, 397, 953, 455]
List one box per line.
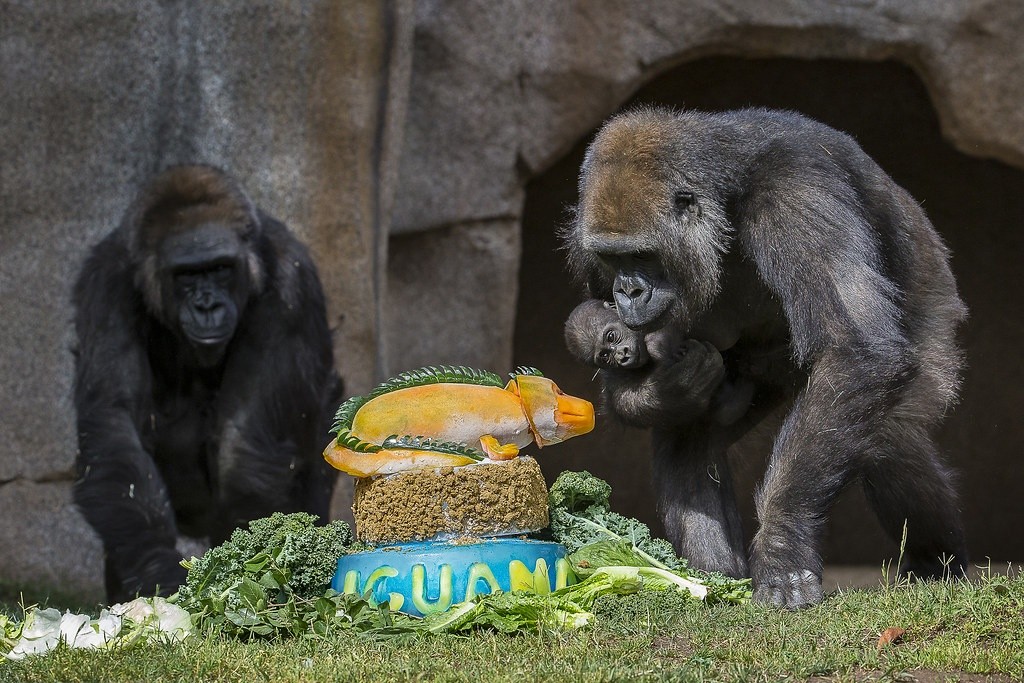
[158, 469, 758, 648]
